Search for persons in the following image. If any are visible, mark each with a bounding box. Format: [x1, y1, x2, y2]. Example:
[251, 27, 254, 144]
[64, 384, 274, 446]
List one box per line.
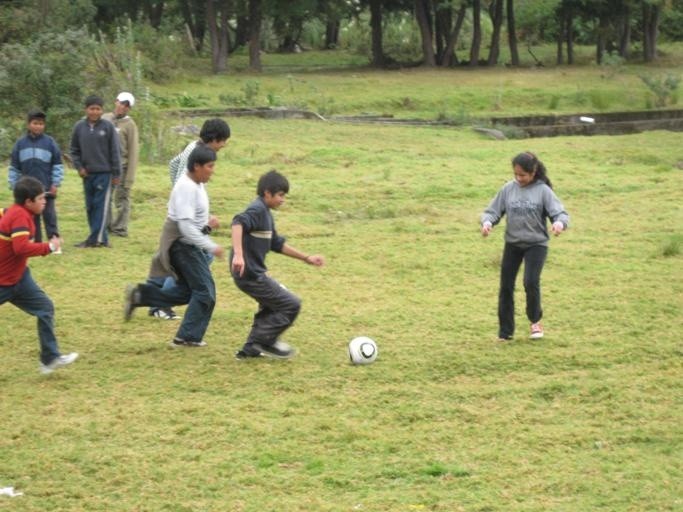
[123, 147, 224, 348]
[9, 112, 64, 255]
[1, 177, 79, 372]
[230, 171, 323, 360]
[99, 92, 139, 235]
[147, 119, 231, 319]
[70, 95, 121, 248]
[481, 151, 569, 343]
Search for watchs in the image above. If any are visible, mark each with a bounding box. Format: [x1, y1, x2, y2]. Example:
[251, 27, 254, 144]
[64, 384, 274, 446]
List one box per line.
[49, 242, 56, 254]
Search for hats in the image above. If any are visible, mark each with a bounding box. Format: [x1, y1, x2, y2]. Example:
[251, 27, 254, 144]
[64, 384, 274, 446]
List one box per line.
[119, 91, 132, 106]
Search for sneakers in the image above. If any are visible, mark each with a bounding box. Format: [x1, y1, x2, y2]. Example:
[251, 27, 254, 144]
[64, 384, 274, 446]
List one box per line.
[122, 282, 138, 320]
[172, 335, 206, 346]
[252, 339, 292, 359]
[527, 322, 541, 337]
[233, 347, 271, 362]
[148, 305, 185, 322]
[40, 352, 79, 370]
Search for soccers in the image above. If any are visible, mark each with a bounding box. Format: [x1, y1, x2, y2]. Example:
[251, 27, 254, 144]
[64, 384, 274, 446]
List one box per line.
[348, 336, 378, 364]
